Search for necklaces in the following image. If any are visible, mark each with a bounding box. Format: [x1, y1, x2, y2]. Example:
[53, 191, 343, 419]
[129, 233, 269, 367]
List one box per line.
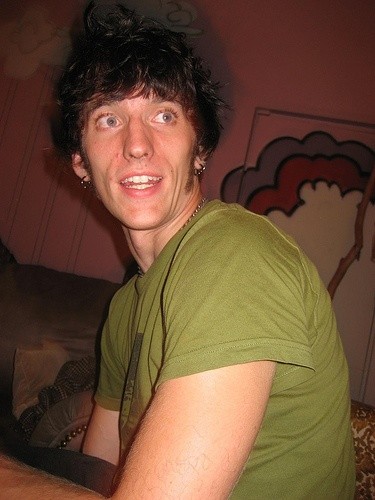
[137, 196, 206, 279]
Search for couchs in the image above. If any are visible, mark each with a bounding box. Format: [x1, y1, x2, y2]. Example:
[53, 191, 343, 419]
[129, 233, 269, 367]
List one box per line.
[1, 240, 374, 499]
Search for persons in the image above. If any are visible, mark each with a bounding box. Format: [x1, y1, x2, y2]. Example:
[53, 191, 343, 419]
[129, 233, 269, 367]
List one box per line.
[0, 1, 356, 500]
